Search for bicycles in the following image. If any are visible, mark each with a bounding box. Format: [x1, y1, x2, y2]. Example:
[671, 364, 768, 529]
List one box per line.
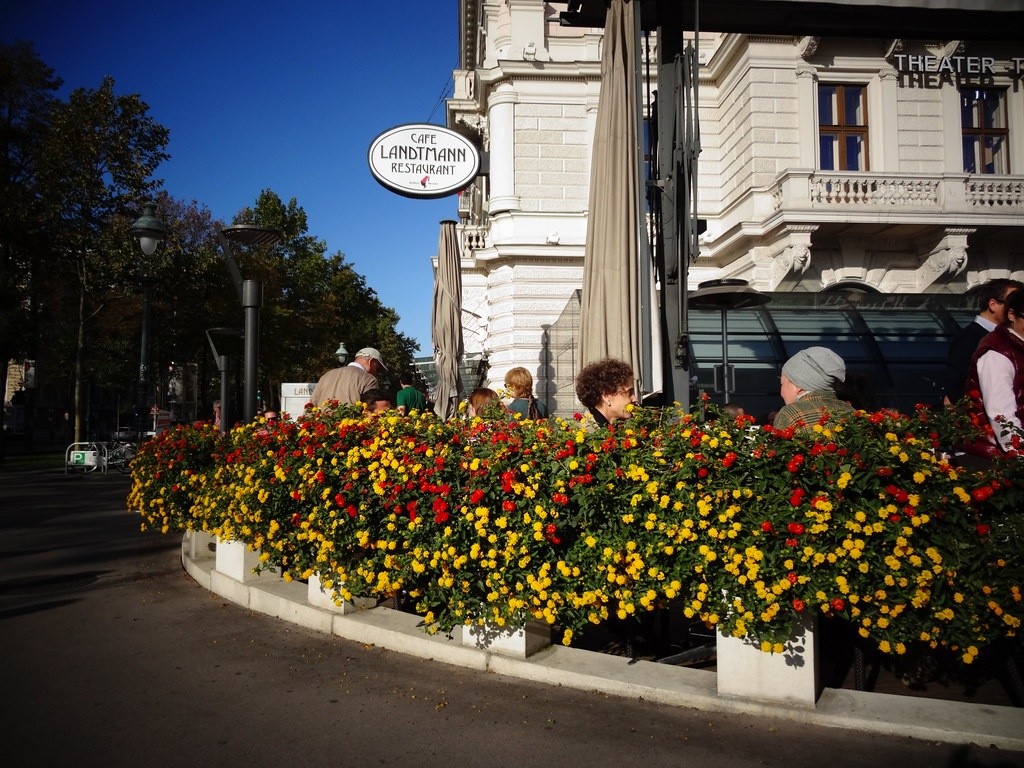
[75, 439, 140, 473]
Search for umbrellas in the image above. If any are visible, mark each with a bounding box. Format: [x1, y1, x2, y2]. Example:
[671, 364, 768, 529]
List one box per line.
[577, 1, 663, 406]
[431, 220, 465, 420]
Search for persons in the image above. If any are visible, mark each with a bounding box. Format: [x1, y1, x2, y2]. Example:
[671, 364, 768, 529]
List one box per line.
[723, 403, 744, 418]
[949, 278, 1024, 407]
[207, 400, 221, 430]
[466, 388, 500, 420]
[774, 345, 855, 433]
[309, 347, 388, 414]
[575, 359, 638, 432]
[504, 367, 548, 421]
[396, 372, 426, 415]
[966, 288, 1024, 461]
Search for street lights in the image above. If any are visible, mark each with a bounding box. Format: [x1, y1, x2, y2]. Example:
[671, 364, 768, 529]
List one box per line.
[128, 202, 172, 471]
[335, 342, 349, 369]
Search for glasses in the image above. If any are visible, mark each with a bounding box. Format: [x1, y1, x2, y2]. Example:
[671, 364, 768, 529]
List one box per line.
[217, 406, 221, 410]
[504, 384, 508, 388]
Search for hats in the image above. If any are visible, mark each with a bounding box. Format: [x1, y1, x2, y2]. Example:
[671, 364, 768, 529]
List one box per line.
[782, 347, 846, 392]
[355, 347, 389, 372]
